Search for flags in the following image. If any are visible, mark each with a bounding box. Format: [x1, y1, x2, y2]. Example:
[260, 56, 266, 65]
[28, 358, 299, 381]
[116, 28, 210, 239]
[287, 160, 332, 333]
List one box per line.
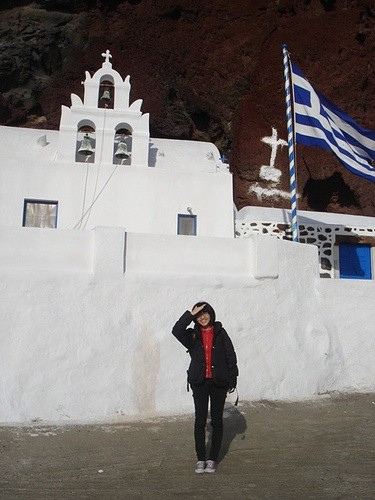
[289, 59, 375, 183]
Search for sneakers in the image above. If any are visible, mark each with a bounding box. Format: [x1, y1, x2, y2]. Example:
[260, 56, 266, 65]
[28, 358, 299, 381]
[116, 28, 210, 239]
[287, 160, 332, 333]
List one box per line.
[204, 460, 216, 473]
[195, 461, 204, 473]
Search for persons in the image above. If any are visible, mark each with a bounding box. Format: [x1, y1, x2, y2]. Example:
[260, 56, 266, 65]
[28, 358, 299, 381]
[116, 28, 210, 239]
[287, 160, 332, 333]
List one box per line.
[172, 301, 239, 474]
